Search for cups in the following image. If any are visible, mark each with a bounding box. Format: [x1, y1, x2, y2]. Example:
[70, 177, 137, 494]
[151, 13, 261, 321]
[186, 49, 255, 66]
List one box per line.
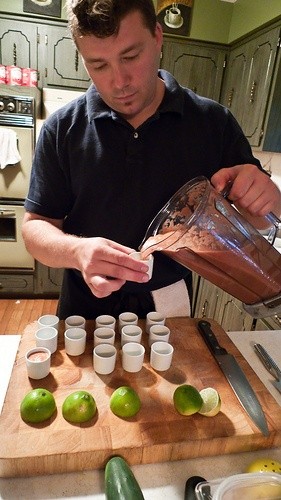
[92, 344, 116, 375]
[38, 314, 60, 332]
[65, 327, 87, 356]
[167, 7, 181, 25]
[120, 325, 143, 348]
[122, 342, 146, 374]
[145, 312, 165, 334]
[149, 323, 170, 346]
[150, 341, 173, 370]
[94, 328, 115, 348]
[118, 312, 138, 336]
[96, 315, 117, 331]
[128, 252, 153, 282]
[35, 326, 58, 355]
[25, 347, 50, 379]
[65, 314, 86, 330]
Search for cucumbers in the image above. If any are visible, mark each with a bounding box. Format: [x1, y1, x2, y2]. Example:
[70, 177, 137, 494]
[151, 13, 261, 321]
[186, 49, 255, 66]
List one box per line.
[104, 457, 144, 500]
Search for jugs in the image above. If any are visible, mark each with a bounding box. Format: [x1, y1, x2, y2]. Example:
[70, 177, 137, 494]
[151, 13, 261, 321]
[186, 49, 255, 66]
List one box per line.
[138, 176, 281, 317]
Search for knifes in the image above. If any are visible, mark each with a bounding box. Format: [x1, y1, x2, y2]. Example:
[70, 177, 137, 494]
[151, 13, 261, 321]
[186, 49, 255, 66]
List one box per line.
[198, 319, 270, 436]
[252, 342, 280, 392]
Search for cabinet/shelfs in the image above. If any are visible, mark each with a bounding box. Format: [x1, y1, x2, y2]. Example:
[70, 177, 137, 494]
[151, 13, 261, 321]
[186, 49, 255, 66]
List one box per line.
[0, 10, 281, 330]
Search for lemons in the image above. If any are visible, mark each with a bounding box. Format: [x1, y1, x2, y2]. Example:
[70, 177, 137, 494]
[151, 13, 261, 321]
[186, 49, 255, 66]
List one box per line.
[62, 391, 97, 423]
[174, 384, 204, 415]
[110, 386, 141, 417]
[197, 388, 221, 417]
[20, 388, 56, 423]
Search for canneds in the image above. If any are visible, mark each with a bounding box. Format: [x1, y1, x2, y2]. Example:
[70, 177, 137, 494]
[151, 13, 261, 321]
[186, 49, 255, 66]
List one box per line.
[0, 63, 39, 88]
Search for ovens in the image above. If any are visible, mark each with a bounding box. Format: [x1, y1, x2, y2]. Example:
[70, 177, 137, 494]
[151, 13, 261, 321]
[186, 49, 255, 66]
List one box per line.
[0, 95, 36, 273]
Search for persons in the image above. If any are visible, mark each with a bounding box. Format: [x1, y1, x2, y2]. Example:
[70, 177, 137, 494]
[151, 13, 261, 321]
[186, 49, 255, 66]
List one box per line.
[20, 0, 281, 318]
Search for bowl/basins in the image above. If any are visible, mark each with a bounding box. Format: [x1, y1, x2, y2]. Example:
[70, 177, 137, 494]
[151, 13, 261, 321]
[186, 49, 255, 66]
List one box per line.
[212, 472, 281, 500]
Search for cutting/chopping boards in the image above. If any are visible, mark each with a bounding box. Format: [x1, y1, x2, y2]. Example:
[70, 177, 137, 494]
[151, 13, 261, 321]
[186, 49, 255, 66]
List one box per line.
[0, 318, 281, 477]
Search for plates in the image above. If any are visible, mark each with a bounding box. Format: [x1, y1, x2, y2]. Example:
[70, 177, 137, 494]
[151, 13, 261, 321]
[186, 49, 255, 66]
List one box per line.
[164, 14, 183, 28]
